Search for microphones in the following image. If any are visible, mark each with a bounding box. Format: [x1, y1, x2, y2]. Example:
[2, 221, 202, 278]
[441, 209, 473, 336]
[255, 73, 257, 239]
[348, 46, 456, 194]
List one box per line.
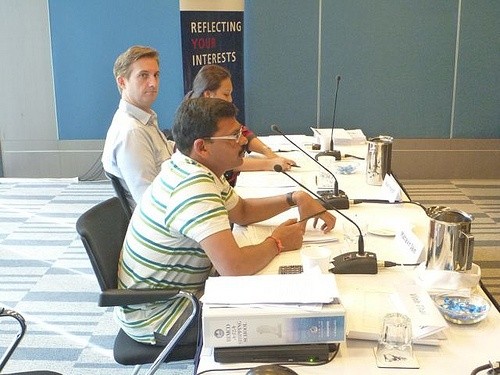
[274, 164, 378, 274]
[314, 75, 342, 160]
[270, 125, 349, 210]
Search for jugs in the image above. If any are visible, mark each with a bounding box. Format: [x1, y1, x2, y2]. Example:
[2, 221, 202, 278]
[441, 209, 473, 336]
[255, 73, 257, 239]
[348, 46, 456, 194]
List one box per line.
[425, 205, 474, 273]
[365, 135, 394, 185]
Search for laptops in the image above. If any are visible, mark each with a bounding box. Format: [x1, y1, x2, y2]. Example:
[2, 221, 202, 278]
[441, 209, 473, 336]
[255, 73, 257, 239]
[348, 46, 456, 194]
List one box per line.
[214, 343, 337, 364]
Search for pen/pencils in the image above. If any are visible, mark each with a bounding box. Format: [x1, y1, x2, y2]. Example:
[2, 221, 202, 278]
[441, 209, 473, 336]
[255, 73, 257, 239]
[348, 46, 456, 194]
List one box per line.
[295, 210, 327, 223]
[289, 165, 301, 167]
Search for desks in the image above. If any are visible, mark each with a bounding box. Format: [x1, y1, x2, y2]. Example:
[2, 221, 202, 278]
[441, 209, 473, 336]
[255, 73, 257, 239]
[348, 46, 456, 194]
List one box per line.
[197, 134, 500, 375]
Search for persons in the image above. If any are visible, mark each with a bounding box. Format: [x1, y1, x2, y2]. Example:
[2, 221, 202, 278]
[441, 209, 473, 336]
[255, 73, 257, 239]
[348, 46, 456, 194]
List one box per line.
[100, 45, 177, 212]
[116, 96, 336, 347]
[184, 65, 297, 186]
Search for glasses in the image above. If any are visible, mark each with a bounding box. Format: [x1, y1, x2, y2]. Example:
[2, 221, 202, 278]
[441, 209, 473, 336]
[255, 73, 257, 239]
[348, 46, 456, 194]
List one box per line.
[201, 125, 242, 143]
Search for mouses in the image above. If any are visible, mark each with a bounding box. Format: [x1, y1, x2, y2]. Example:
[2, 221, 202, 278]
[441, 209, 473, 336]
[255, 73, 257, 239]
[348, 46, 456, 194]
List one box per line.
[245, 364, 298, 375]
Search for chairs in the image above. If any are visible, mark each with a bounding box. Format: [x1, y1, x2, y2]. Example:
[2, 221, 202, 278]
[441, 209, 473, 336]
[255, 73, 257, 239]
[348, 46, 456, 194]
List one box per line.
[76, 199, 201, 374]
[0, 307, 65, 375]
[105, 167, 134, 223]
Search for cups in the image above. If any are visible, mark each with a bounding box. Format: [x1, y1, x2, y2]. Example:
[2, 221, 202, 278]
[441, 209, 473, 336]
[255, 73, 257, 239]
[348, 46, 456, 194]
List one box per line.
[375, 313, 413, 366]
[319, 134, 334, 150]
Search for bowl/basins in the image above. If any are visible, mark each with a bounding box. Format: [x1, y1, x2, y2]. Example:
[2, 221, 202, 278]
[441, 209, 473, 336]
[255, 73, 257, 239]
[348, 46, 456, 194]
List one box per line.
[433, 291, 491, 325]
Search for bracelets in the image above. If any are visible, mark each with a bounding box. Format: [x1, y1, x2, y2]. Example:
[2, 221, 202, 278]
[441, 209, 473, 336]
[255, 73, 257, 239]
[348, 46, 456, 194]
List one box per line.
[286, 190, 297, 208]
[262, 147, 272, 155]
[264, 235, 282, 255]
